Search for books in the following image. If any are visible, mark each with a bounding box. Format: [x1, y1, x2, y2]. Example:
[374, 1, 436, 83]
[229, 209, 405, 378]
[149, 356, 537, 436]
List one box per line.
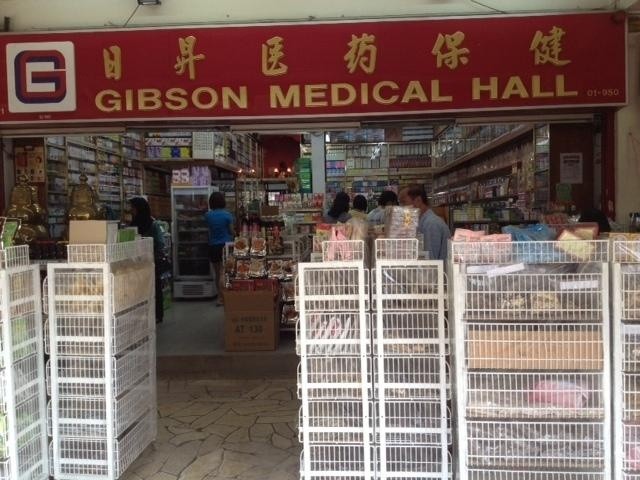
[485, 262, 530, 277]
[558, 277, 600, 290]
[466, 262, 500, 276]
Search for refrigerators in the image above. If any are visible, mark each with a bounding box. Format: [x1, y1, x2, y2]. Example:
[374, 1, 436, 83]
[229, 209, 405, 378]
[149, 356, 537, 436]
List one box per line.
[170, 184, 219, 299]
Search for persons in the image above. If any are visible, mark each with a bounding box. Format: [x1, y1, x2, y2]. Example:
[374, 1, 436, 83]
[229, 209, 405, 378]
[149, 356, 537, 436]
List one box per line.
[324, 190, 353, 226]
[367, 190, 399, 223]
[398, 185, 452, 282]
[123, 197, 169, 326]
[348, 194, 369, 233]
[204, 191, 237, 306]
